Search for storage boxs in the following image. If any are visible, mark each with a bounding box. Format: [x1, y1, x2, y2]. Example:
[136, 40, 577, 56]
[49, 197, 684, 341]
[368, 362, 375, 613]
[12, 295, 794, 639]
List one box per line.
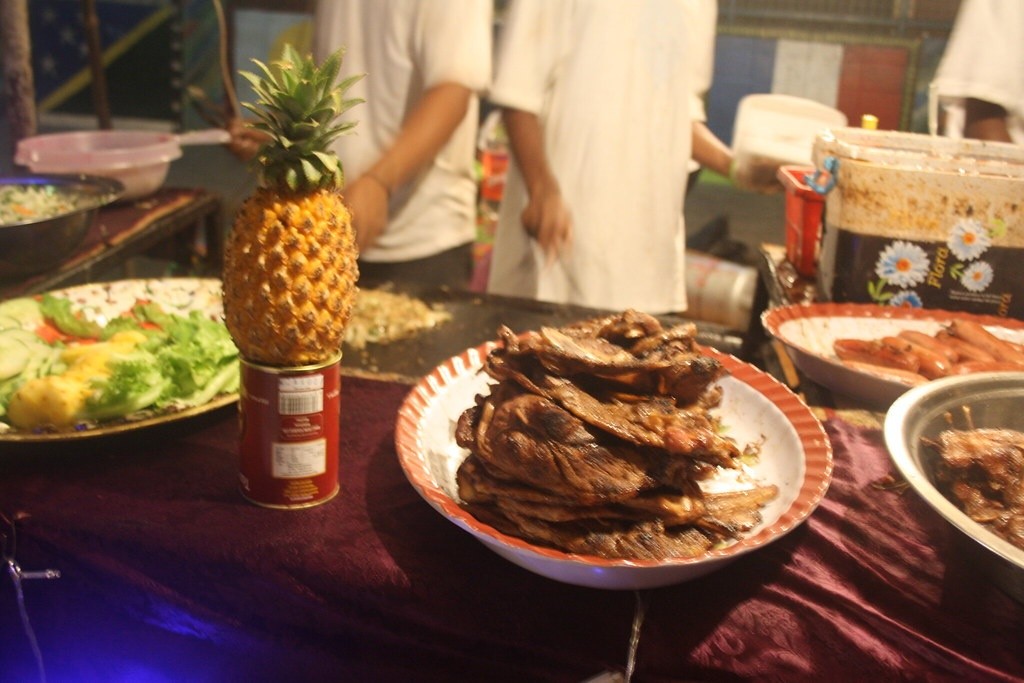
[803, 126, 1024, 321]
[777, 164, 828, 279]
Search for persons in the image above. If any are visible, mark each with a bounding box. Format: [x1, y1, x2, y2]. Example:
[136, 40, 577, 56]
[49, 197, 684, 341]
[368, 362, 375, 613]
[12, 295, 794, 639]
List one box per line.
[485, 0, 803, 317]
[224, 0, 490, 295]
[928, 0, 1024, 149]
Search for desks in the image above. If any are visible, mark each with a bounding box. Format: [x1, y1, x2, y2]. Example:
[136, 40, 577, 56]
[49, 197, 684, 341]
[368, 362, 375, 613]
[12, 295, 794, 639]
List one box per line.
[0, 263, 1024, 683]
[0, 169, 226, 300]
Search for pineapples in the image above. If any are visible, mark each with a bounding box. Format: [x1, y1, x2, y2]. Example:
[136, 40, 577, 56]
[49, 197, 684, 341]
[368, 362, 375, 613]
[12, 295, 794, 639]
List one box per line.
[222, 45, 367, 365]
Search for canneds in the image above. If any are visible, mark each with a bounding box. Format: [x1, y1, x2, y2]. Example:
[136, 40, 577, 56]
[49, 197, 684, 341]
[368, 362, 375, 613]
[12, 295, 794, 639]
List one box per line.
[238, 348, 342, 510]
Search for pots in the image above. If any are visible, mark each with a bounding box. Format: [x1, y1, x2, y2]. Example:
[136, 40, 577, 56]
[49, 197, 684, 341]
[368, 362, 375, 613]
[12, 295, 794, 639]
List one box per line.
[15, 129, 233, 204]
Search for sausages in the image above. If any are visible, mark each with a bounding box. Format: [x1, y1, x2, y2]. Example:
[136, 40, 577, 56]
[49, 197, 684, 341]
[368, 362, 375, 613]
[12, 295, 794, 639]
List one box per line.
[832, 318, 1024, 390]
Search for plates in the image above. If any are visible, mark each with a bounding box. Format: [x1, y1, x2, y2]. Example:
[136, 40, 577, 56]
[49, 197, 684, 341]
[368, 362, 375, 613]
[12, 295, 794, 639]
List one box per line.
[760, 301, 1024, 410]
[393, 338, 836, 590]
[0, 276, 242, 442]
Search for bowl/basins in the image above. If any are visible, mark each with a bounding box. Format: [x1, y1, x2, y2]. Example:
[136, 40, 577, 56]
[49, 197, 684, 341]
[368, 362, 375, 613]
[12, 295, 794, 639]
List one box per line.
[882, 371, 1024, 605]
[0, 173, 129, 282]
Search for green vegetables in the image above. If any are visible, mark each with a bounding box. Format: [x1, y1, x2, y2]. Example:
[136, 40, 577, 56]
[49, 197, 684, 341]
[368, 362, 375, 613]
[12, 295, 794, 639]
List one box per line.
[0, 292, 239, 420]
